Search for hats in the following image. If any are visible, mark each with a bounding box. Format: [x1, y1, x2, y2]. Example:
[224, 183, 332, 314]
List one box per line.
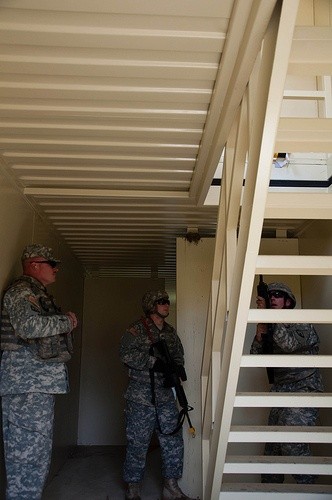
[22, 243, 62, 263]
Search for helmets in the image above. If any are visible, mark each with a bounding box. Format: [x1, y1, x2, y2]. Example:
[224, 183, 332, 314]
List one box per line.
[267, 282, 296, 309]
[143, 289, 169, 314]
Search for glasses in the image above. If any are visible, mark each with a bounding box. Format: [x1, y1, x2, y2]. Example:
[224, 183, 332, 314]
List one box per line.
[30, 260, 56, 268]
[268, 291, 285, 299]
[156, 298, 170, 306]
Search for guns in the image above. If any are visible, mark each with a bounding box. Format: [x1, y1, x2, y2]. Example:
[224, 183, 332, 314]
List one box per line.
[150, 339, 194, 430]
[256, 275, 282, 385]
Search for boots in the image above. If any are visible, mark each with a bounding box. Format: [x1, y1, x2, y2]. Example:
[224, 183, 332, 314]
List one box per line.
[125, 482, 142, 500]
[162, 478, 194, 500]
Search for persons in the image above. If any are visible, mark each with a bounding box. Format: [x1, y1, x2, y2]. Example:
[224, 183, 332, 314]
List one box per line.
[0, 244, 78, 500]
[117, 290, 191, 500]
[249, 282, 323, 484]
